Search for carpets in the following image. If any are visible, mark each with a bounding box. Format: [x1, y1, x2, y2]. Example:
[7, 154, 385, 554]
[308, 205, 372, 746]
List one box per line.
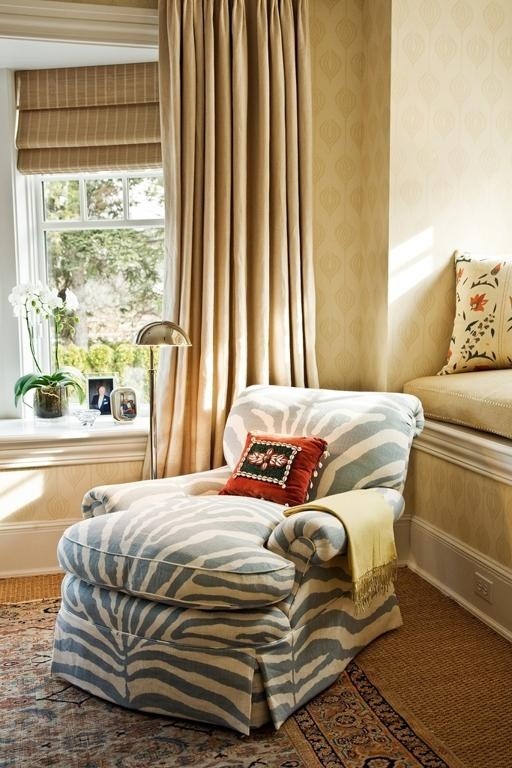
[0, 597, 470, 767]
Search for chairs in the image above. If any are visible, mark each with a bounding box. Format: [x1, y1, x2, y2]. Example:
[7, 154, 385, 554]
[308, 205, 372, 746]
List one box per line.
[52, 383, 425, 736]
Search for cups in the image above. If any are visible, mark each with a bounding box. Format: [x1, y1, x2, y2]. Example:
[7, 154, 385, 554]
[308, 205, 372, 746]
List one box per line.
[76, 408, 101, 428]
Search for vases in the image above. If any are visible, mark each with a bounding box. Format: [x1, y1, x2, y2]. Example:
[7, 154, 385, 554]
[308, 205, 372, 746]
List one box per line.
[35, 385, 69, 419]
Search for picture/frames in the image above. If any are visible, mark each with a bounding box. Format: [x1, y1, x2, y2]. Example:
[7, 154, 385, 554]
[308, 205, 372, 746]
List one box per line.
[87, 376, 116, 417]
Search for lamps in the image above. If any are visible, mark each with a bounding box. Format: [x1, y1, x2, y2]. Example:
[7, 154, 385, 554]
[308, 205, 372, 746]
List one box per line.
[135, 320, 193, 479]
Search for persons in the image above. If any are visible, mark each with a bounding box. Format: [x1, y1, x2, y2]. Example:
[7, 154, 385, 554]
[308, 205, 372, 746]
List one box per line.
[92, 385, 112, 415]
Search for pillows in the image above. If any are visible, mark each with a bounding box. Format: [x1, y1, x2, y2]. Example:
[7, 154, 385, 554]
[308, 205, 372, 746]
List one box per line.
[434, 249, 512, 376]
[221, 428, 328, 507]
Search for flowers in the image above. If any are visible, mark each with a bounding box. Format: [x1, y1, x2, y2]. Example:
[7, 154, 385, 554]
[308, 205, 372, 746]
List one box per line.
[2, 276, 88, 407]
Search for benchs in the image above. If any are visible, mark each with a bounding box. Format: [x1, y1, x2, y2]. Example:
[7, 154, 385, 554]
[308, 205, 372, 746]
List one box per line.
[405, 367, 512, 643]
